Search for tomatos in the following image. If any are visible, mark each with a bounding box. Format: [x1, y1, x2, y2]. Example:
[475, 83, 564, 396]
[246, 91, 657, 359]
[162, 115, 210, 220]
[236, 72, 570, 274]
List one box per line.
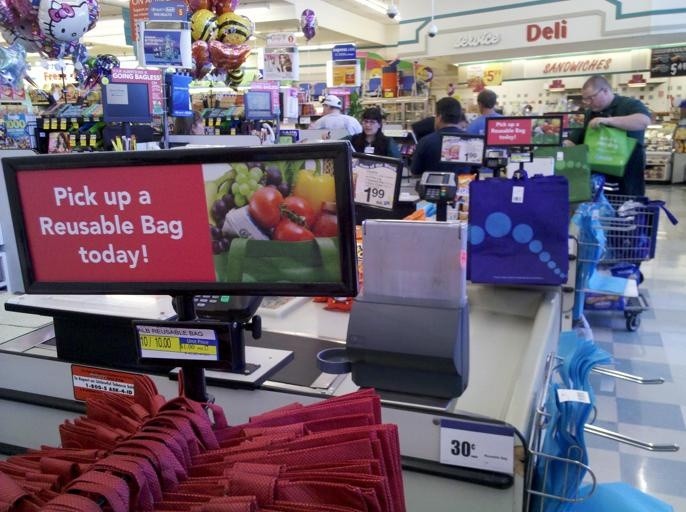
[250, 187, 339, 242]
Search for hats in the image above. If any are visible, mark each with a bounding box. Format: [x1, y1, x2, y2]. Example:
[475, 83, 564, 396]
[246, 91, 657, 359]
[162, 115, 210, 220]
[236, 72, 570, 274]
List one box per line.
[318, 94, 343, 108]
[362, 107, 384, 121]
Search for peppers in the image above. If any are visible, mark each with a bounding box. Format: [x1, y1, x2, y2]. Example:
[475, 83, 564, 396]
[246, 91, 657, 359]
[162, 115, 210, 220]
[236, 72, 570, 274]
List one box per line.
[293, 158, 335, 213]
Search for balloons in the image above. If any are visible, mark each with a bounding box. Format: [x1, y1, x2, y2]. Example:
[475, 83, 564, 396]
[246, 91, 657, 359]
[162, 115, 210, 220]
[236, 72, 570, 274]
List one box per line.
[0, 1, 121, 93]
[301, 9, 319, 42]
[186, 2, 255, 92]
[416, 63, 484, 109]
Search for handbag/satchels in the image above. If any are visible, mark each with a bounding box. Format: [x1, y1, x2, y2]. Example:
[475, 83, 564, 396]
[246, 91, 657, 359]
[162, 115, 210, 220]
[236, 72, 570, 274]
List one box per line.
[544, 334, 673, 511]
[535, 145, 594, 202]
[465, 172, 570, 287]
[584, 125, 638, 177]
[626, 198, 664, 257]
[0, 391, 407, 512]
[211, 235, 344, 285]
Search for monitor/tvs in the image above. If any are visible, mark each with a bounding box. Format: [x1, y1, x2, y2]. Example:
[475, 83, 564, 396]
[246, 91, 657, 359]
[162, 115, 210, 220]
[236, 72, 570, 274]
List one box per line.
[102, 82, 151, 123]
[244, 90, 273, 121]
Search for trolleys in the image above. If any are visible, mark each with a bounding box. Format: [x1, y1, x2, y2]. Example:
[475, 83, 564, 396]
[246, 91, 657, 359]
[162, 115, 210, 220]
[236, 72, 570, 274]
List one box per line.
[581, 175, 665, 335]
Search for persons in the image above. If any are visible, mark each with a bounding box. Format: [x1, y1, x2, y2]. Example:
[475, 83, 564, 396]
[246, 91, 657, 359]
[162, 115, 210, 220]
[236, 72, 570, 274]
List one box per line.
[407, 96, 476, 182]
[562, 74, 654, 271]
[275, 54, 293, 73]
[249, 122, 275, 145]
[337, 108, 405, 161]
[306, 95, 365, 141]
[464, 91, 506, 137]
[158, 111, 203, 150]
[406, 113, 470, 144]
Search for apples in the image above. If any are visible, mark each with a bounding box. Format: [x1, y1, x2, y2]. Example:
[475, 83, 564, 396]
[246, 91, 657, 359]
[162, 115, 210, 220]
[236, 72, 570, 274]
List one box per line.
[535, 118, 560, 137]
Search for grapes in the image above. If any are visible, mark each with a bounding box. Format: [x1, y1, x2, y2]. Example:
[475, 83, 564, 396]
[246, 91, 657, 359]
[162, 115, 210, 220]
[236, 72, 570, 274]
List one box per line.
[210, 163, 288, 255]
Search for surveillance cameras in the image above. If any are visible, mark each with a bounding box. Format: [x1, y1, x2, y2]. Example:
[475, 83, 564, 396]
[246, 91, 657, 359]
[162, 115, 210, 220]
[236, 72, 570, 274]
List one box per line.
[386, 5, 397, 20]
[428, 24, 436, 36]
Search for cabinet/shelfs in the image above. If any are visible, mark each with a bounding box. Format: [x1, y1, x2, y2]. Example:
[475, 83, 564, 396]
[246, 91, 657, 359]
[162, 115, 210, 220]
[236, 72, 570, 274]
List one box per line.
[639, 126, 686, 184]
[358, 96, 436, 127]
